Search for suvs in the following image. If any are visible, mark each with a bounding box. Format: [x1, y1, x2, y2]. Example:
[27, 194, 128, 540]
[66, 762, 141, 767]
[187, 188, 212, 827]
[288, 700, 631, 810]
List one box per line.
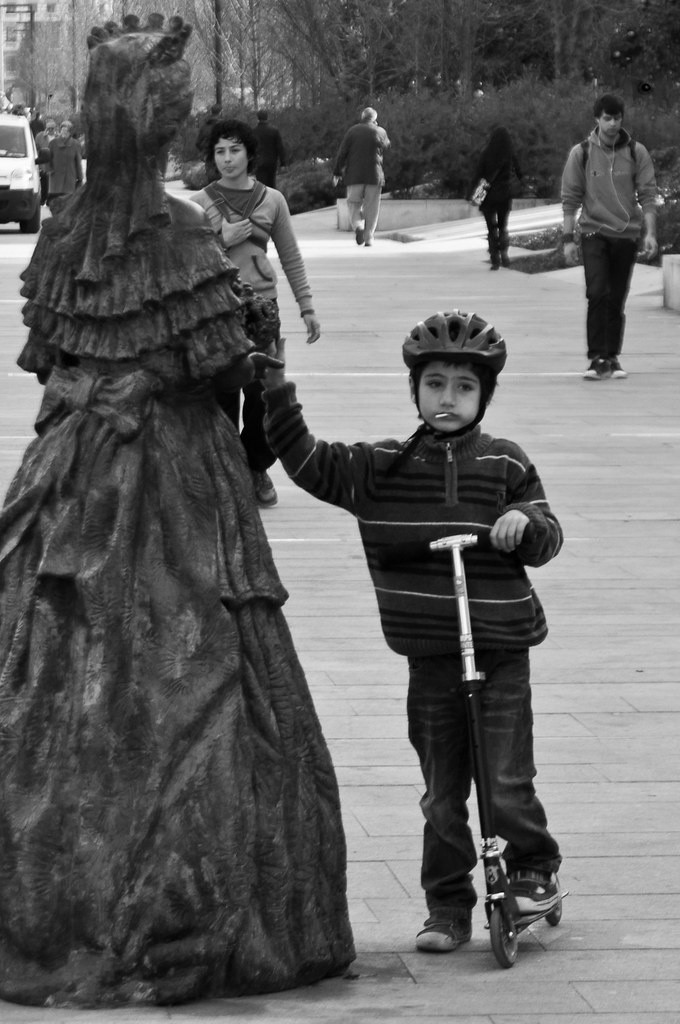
[0, 114, 52, 233]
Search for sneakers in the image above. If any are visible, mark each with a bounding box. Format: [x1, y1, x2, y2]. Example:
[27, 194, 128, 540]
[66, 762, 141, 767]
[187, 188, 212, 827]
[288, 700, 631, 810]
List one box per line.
[415, 916, 473, 952]
[508, 874, 559, 913]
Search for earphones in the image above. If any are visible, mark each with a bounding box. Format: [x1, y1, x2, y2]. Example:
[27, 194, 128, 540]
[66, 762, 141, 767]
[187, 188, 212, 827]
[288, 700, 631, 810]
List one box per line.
[596, 119, 599, 123]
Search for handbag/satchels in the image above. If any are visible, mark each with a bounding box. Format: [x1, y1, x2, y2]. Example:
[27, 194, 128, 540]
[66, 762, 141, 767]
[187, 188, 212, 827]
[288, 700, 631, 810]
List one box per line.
[470, 177, 490, 206]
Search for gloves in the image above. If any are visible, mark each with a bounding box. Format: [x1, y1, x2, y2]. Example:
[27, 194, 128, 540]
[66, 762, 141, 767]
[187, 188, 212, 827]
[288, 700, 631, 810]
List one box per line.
[238, 352, 285, 380]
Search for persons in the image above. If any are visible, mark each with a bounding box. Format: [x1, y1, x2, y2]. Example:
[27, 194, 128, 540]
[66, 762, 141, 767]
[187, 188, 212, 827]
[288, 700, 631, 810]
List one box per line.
[0, 13, 358, 1010]
[251, 110, 286, 188]
[44, 121, 83, 195]
[195, 104, 222, 182]
[29, 112, 45, 139]
[560, 94, 658, 381]
[257, 310, 564, 953]
[190, 119, 321, 507]
[466, 127, 522, 270]
[35, 119, 58, 206]
[332, 107, 391, 246]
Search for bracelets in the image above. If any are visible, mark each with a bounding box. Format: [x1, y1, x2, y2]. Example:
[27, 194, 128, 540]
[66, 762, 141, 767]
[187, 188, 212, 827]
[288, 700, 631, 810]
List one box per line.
[562, 233, 573, 243]
[300, 309, 315, 317]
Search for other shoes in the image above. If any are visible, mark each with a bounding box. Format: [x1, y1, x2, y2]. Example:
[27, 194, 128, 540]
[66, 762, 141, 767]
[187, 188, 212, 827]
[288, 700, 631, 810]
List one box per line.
[249, 470, 277, 510]
[609, 354, 627, 379]
[358, 226, 364, 245]
[583, 357, 611, 378]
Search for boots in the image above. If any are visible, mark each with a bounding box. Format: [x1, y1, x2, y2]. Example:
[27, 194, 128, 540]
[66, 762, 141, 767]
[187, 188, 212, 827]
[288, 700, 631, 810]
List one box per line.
[487, 234, 500, 271]
[499, 232, 510, 267]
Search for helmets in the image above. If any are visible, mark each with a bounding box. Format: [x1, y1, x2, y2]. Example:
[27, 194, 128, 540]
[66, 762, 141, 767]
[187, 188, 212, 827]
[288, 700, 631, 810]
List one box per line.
[403, 309, 507, 375]
